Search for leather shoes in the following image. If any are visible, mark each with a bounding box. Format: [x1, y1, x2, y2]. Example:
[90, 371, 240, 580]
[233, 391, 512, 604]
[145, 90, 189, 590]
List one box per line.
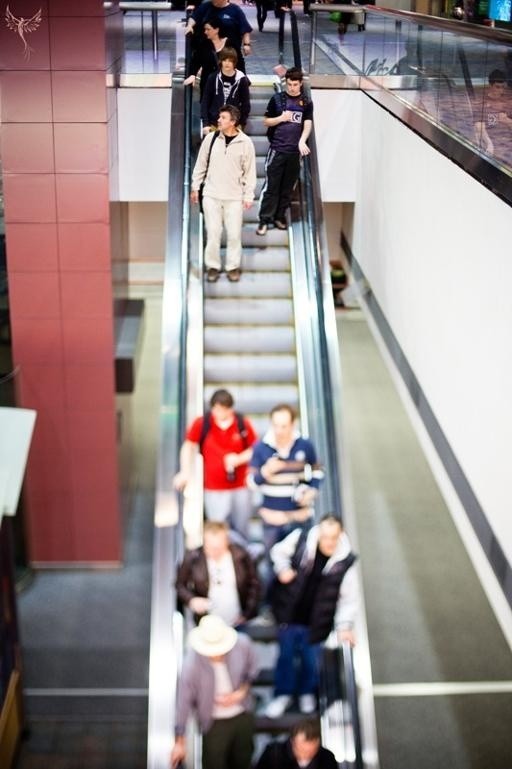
[208, 269, 239, 282]
[256, 220, 287, 235]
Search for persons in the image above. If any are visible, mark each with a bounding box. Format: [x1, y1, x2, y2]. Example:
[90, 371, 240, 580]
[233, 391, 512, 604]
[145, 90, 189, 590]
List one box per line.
[256, 66, 313, 236]
[202, 48, 252, 141]
[183, 17, 234, 106]
[186, 0, 210, 13]
[474, 68, 511, 167]
[302, 1, 376, 34]
[414, 48, 481, 149]
[253, 0, 268, 32]
[399, 36, 426, 90]
[188, 104, 258, 281]
[171, 386, 359, 769]
[184, 0, 253, 75]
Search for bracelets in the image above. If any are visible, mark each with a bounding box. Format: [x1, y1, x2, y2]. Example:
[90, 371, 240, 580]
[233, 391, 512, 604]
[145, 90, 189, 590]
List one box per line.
[244, 43, 251, 46]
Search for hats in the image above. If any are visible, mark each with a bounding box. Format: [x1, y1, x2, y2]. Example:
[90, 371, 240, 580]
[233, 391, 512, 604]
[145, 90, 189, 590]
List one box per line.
[188, 615, 237, 656]
